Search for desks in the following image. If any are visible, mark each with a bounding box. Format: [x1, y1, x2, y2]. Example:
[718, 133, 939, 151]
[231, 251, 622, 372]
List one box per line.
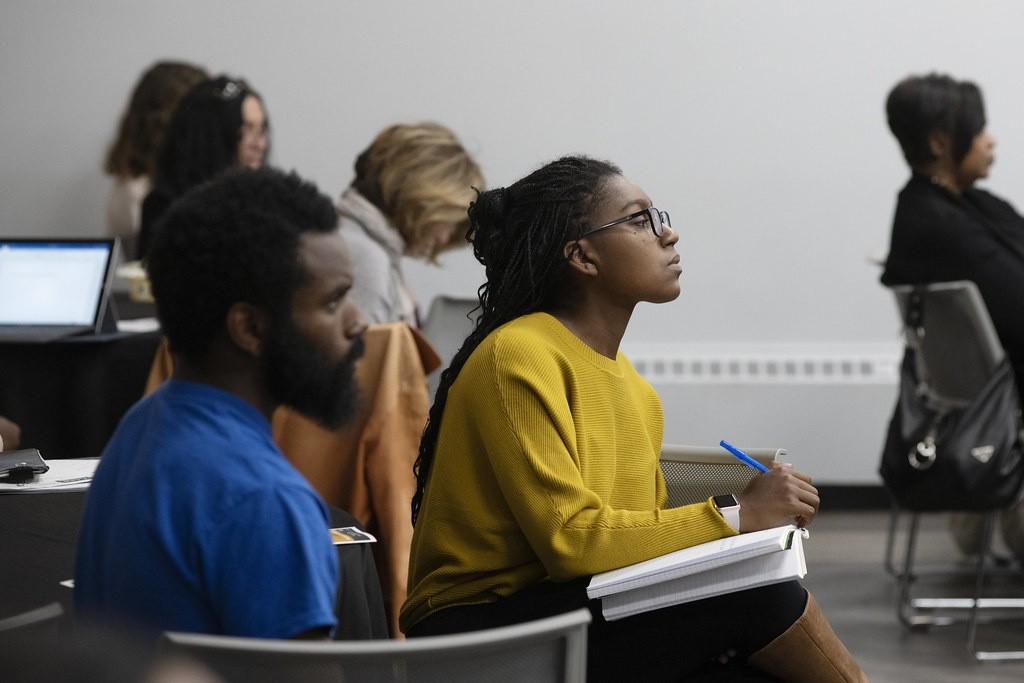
[0, 453, 388, 683]
[0, 235, 160, 458]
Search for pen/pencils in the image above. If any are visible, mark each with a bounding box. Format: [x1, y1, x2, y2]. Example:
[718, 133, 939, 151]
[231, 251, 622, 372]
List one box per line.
[719, 439, 770, 474]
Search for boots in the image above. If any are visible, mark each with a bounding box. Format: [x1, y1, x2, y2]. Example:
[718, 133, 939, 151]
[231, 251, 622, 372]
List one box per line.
[747, 587, 863, 683]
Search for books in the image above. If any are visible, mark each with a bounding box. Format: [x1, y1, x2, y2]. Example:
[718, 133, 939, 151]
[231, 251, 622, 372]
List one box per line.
[587, 523, 810, 620]
[0, 449, 49, 480]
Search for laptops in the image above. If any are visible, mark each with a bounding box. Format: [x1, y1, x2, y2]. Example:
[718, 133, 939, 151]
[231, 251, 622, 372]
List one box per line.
[0, 236, 120, 343]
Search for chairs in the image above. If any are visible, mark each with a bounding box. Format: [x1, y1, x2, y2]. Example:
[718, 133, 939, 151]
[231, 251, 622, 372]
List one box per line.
[170, 611, 595, 683]
[646, 447, 800, 510]
[871, 280, 1024, 668]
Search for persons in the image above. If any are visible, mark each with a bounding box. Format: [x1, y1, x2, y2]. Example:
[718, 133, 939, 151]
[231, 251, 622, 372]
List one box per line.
[0, 415, 21, 453]
[95, 57, 271, 262]
[883, 73, 1024, 419]
[63, 162, 369, 641]
[315, 122, 483, 329]
[391, 159, 872, 683]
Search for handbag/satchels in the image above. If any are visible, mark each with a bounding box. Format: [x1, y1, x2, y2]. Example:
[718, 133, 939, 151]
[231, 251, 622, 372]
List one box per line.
[879, 284, 1023, 513]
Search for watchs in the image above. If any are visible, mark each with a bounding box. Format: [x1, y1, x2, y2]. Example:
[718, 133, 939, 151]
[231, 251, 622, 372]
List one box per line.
[711, 492, 741, 531]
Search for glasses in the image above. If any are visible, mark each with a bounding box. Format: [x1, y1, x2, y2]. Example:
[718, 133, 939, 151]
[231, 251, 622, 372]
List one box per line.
[575, 207, 671, 239]
